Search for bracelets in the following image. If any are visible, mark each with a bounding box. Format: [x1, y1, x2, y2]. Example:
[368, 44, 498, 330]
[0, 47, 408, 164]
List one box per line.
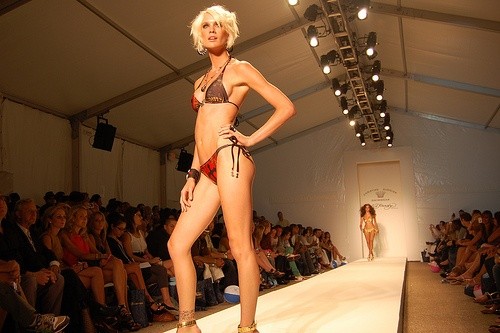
[185, 168, 201, 187]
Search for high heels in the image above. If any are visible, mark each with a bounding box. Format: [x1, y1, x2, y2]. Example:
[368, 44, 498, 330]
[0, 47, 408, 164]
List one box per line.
[147, 300, 166, 314]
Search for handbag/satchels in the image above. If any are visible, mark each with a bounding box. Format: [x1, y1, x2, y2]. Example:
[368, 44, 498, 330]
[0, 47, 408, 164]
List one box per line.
[428, 261, 442, 272]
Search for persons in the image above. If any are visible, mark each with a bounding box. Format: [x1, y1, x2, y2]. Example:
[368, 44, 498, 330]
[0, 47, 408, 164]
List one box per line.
[423, 208, 500, 333]
[0, 191, 347, 333]
[166, 6, 295, 333]
[359, 204, 379, 260]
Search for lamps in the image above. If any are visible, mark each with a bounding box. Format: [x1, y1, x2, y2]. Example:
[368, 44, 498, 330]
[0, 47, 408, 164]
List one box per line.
[354, 0, 371, 20]
[321, 50, 339, 74]
[374, 80, 384, 101]
[385, 127, 392, 140]
[364, 32, 376, 56]
[387, 137, 394, 147]
[383, 113, 390, 130]
[371, 59, 381, 82]
[307, 25, 319, 47]
[331, 78, 366, 147]
[288, 0, 298, 5]
[378, 100, 387, 117]
[303, 4, 320, 20]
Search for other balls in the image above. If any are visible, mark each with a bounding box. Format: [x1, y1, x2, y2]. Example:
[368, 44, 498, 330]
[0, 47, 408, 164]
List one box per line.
[330, 260, 338, 269]
[224, 284, 240, 302]
[341, 260, 348, 266]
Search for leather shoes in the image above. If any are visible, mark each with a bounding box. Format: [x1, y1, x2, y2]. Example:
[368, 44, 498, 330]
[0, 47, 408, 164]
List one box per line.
[92, 304, 124, 321]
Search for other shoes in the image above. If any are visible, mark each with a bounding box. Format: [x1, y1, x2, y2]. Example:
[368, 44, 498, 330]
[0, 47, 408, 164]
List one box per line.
[238, 322, 259, 333]
[312, 271, 321, 275]
[176, 319, 202, 333]
[161, 302, 179, 311]
[288, 253, 300, 261]
[121, 317, 143, 331]
[94, 320, 118, 333]
[431, 258, 500, 333]
[295, 276, 308, 281]
[304, 274, 312, 277]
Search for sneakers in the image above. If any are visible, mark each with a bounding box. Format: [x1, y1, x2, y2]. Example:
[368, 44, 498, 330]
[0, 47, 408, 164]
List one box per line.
[27, 313, 70, 333]
